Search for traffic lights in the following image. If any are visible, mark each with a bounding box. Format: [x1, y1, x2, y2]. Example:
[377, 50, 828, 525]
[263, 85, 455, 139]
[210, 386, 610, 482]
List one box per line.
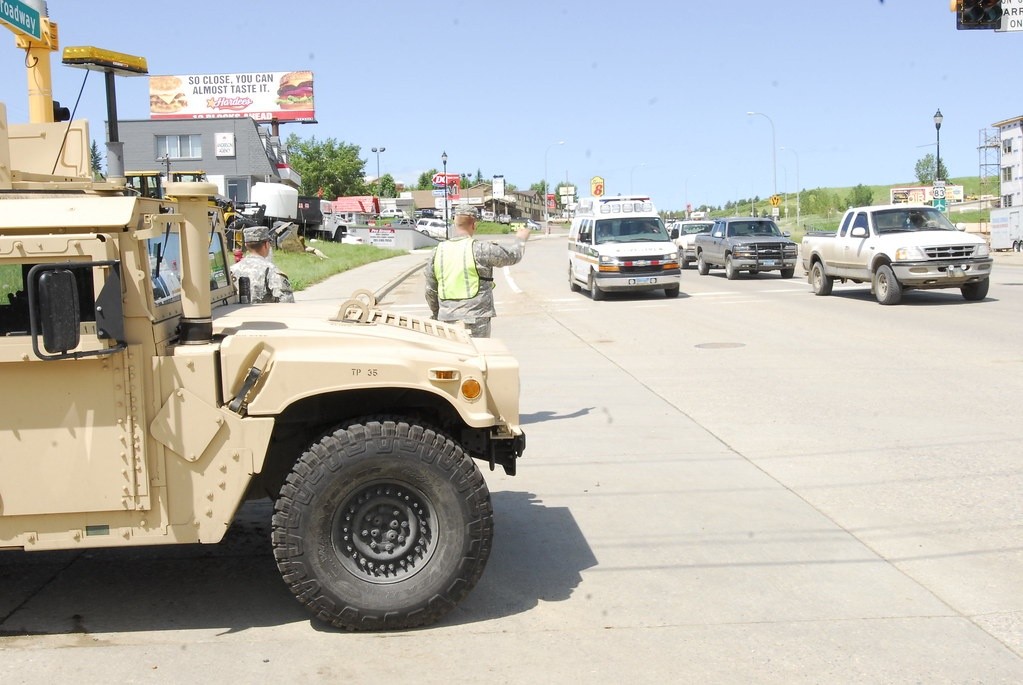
[956, 0, 1003, 30]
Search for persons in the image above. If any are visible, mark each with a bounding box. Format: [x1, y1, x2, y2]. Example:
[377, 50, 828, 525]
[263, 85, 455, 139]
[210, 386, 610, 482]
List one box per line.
[229, 226, 295, 304]
[643, 222, 660, 233]
[424, 204, 532, 337]
[600, 224, 611, 237]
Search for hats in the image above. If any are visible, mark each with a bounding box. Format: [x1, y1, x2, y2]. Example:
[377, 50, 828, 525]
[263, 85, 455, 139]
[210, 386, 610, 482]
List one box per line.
[455, 204, 478, 219]
[243, 226, 273, 242]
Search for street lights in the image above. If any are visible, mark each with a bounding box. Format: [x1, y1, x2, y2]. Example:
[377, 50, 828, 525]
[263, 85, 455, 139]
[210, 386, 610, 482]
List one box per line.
[460, 173, 472, 204]
[371, 147, 385, 220]
[441, 150, 448, 240]
[933, 108, 944, 179]
[545, 141, 565, 234]
[780, 146, 800, 226]
[747, 112, 777, 225]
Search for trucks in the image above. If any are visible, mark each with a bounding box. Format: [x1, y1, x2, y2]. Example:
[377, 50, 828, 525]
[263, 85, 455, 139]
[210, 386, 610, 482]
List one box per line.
[989, 205, 1023, 253]
[414, 209, 442, 218]
[497, 214, 512, 225]
[264, 196, 349, 242]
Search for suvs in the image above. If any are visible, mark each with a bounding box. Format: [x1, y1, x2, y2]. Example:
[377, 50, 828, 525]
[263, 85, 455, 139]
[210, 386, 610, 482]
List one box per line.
[0, 47, 527, 635]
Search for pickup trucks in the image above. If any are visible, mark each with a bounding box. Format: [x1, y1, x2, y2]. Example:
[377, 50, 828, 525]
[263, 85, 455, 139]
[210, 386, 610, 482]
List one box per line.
[670, 217, 797, 280]
[802, 203, 993, 305]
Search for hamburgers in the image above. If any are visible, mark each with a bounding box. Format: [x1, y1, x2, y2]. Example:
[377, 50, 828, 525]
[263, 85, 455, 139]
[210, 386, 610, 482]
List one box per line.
[149, 76, 188, 113]
[276, 71, 314, 110]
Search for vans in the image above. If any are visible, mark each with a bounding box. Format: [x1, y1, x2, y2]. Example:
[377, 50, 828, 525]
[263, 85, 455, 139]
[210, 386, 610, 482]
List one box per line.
[380, 208, 403, 219]
[482, 212, 496, 222]
[568, 196, 682, 301]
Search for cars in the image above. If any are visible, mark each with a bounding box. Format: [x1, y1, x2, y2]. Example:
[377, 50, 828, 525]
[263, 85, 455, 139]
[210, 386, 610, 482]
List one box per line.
[527, 219, 542, 231]
[416, 218, 450, 238]
[547, 215, 569, 223]
